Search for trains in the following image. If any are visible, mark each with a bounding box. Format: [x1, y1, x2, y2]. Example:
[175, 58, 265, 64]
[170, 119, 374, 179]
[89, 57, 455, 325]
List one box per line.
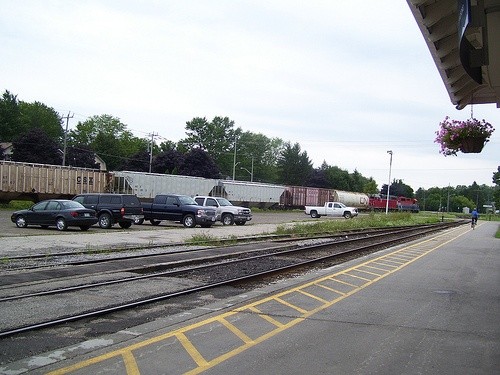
[0, 161, 420, 214]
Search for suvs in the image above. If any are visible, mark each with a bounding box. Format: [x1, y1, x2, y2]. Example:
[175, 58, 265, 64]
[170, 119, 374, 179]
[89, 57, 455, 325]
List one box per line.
[72, 193, 144, 229]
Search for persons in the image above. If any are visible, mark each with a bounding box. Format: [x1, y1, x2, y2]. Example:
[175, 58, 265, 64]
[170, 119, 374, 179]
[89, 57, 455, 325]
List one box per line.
[471, 208, 478, 227]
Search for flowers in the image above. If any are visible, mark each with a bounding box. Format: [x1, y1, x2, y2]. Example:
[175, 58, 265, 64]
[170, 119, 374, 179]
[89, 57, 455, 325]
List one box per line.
[434, 115, 496, 157]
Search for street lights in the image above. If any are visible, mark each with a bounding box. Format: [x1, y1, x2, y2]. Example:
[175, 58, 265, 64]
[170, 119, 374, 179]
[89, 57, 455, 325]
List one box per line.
[386, 150, 393, 214]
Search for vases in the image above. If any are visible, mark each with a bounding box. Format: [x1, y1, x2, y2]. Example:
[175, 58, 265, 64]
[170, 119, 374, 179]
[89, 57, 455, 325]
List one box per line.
[460, 137, 485, 153]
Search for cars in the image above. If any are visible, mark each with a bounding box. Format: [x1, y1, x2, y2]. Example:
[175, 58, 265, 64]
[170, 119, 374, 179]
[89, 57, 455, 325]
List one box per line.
[10, 199, 98, 232]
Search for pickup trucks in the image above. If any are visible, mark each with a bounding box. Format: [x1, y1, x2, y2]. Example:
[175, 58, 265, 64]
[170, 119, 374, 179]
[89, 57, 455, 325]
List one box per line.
[304, 202, 359, 219]
[190, 196, 253, 226]
[133, 194, 216, 229]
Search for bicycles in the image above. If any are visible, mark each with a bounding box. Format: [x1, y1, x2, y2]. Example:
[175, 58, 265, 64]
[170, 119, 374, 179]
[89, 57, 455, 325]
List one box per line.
[472, 216, 479, 230]
